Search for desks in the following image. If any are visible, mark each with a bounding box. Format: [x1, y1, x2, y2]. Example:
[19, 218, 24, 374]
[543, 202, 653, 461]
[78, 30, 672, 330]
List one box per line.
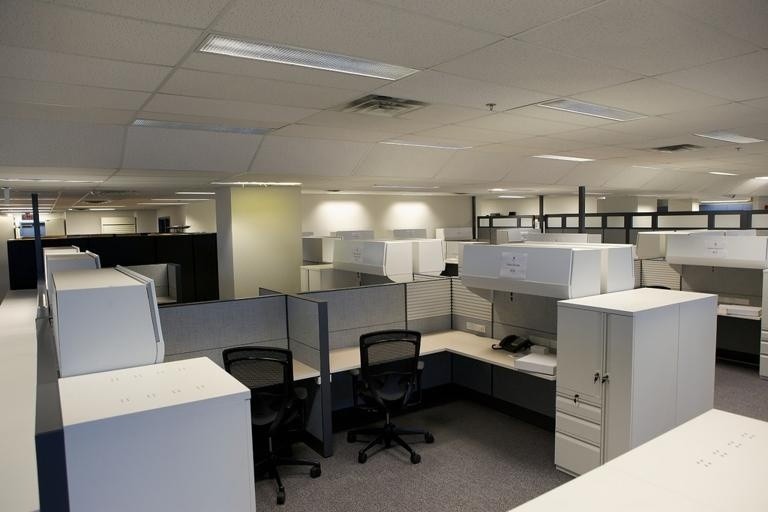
[329, 328, 558, 458]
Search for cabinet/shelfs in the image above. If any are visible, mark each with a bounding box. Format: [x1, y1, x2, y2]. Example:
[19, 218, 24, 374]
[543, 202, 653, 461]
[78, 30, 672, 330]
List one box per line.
[554, 288, 717, 478]
[59, 357, 255, 511]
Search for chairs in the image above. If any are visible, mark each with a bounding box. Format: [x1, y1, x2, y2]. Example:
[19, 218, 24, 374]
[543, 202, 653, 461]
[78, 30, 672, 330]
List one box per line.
[223, 346, 322, 505]
[348, 333, 436, 463]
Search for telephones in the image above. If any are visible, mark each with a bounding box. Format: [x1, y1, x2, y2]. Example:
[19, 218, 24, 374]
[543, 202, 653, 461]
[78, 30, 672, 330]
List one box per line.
[491, 333, 530, 354]
